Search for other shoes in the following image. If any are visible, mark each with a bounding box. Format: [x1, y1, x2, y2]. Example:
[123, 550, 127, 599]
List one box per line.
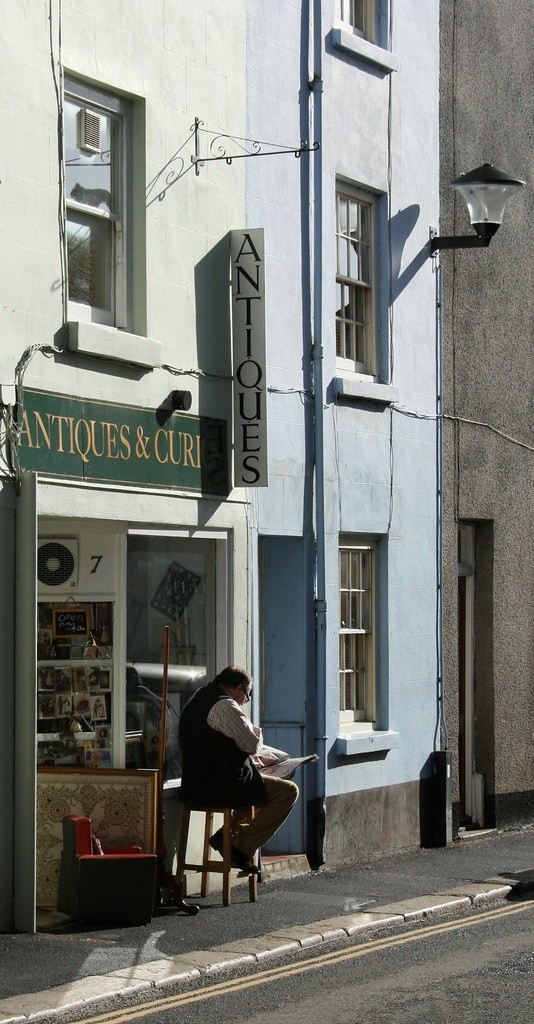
[231, 843, 261, 873]
[208, 831, 224, 858]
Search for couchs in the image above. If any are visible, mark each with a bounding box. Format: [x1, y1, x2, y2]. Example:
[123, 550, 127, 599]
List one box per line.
[56, 815, 157, 927]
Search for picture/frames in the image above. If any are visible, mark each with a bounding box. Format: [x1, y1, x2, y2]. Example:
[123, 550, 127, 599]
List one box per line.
[38, 732, 97, 766]
[96, 668, 113, 693]
[125, 730, 146, 769]
[126, 699, 148, 740]
[36, 765, 159, 912]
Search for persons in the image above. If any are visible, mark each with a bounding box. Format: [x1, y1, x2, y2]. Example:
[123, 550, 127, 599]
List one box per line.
[177, 664, 300, 875]
[124, 664, 172, 752]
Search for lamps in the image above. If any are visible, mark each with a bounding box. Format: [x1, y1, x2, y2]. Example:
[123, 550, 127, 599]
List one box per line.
[429, 163, 527, 258]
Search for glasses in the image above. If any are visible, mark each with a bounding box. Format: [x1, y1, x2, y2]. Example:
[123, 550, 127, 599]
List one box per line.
[243, 688, 250, 703]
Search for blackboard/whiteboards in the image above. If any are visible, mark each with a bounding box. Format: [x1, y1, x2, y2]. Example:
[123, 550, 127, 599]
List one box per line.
[53, 608, 90, 638]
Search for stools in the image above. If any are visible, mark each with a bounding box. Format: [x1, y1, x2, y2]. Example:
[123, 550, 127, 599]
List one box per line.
[176, 804, 258, 908]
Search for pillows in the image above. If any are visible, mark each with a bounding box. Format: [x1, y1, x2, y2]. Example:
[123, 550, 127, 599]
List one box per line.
[92, 835, 104, 855]
